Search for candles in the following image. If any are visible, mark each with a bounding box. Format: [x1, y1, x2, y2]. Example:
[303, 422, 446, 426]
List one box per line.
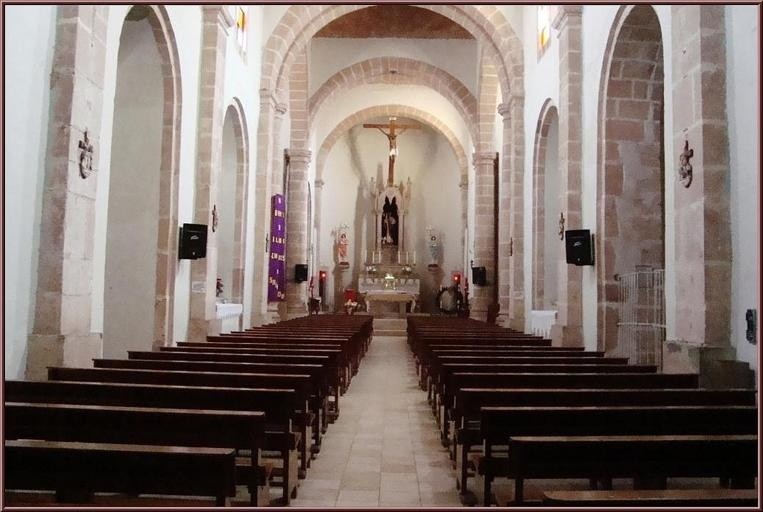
[398, 251, 401, 264]
[406, 251, 408, 264]
[365, 249, 367, 262]
[414, 251, 416, 264]
[372, 251, 374, 263]
[379, 250, 381, 264]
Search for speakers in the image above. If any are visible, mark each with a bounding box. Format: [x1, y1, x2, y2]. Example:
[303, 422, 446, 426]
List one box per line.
[472, 266, 486, 285]
[180, 223, 207, 260]
[564, 228, 592, 266]
[295, 264, 308, 281]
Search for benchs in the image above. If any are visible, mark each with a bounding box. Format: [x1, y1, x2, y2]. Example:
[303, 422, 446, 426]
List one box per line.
[4, 313, 374, 507]
[407, 313, 758, 508]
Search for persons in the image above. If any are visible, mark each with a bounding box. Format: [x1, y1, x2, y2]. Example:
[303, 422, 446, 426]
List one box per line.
[430, 235, 440, 260]
[338, 233, 349, 260]
[558, 211, 567, 239]
[376, 126, 407, 162]
[211, 203, 218, 231]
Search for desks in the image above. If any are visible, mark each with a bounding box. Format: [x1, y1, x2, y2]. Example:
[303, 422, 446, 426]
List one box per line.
[363, 292, 412, 318]
